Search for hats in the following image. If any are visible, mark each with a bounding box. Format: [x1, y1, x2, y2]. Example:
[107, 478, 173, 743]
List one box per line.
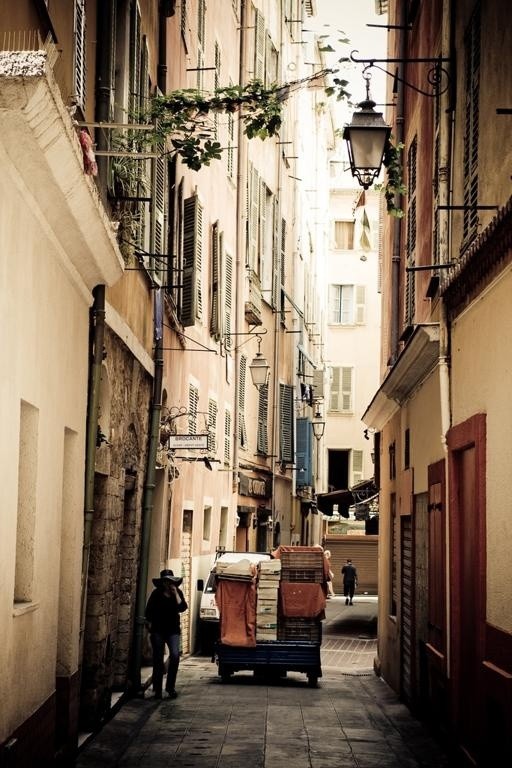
[152, 569, 182, 588]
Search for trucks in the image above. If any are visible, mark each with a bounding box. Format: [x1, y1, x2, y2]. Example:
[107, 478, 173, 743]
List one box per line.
[212, 543, 327, 691]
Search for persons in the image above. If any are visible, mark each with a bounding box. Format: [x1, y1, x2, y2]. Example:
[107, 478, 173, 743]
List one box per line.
[340, 557, 359, 606]
[324, 547, 335, 599]
[144, 569, 188, 699]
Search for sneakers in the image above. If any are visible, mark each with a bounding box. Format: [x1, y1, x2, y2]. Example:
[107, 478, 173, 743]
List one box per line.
[345, 598, 352, 605]
[155, 689, 162, 699]
[166, 687, 178, 699]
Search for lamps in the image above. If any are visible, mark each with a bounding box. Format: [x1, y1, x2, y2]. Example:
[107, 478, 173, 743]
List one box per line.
[223, 327, 270, 392]
[342, 48, 452, 192]
[310, 402, 326, 441]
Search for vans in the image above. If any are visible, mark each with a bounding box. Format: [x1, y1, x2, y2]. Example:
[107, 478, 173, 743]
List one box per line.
[197, 547, 275, 633]
